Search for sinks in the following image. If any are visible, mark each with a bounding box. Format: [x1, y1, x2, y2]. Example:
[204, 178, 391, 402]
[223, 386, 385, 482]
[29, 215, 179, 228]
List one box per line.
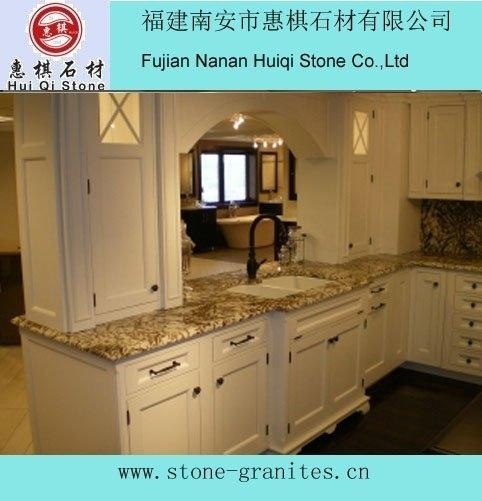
[229, 274, 335, 300]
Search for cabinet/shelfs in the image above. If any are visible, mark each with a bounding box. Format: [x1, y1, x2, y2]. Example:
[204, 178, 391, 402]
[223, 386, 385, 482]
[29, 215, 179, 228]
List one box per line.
[444, 269, 482, 386]
[353, 262, 408, 389]
[297, 96, 375, 263]
[12, 91, 161, 331]
[409, 265, 449, 379]
[10, 316, 271, 455]
[407, 93, 481, 200]
[273, 284, 371, 455]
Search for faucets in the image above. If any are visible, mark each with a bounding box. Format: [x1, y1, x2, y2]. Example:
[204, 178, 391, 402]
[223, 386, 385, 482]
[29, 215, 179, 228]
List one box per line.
[245, 212, 283, 280]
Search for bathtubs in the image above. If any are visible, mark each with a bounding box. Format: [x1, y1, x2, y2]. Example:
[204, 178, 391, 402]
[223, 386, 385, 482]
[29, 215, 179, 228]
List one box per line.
[216, 214, 280, 249]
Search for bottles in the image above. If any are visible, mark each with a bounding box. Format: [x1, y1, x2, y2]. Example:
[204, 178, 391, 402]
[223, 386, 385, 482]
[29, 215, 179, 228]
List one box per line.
[278, 245, 291, 265]
[286, 225, 304, 265]
[301, 233, 310, 265]
[181, 220, 191, 275]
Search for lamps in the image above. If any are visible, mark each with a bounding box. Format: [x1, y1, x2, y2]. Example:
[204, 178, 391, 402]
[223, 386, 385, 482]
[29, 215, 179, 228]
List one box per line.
[247, 131, 284, 151]
[226, 111, 249, 129]
